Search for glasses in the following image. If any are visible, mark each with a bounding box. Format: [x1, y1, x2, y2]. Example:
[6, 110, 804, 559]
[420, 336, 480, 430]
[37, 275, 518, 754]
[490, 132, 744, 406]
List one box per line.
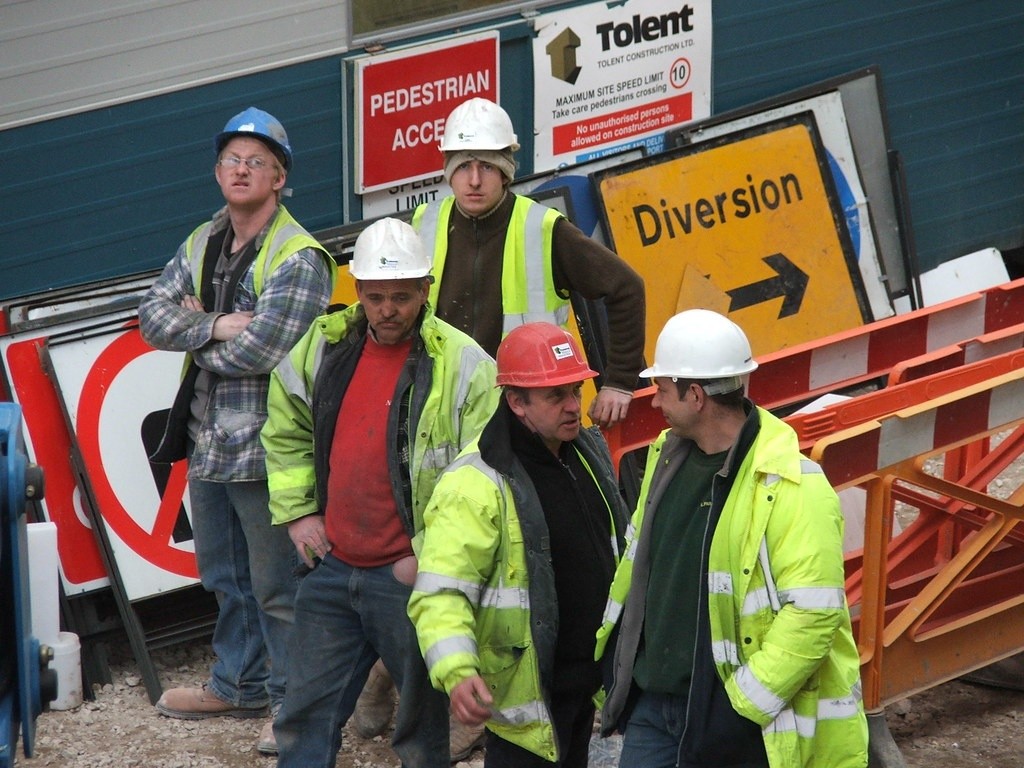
[216, 157, 276, 171]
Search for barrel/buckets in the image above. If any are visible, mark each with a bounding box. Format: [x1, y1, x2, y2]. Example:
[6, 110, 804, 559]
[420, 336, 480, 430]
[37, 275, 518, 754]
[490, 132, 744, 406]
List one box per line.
[31, 632, 83, 709]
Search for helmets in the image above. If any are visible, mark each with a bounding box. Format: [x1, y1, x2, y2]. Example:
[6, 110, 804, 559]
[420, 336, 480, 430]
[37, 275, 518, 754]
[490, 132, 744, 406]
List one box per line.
[213, 107, 294, 174]
[639, 309, 758, 379]
[349, 217, 436, 284]
[438, 98, 520, 151]
[493, 322, 600, 388]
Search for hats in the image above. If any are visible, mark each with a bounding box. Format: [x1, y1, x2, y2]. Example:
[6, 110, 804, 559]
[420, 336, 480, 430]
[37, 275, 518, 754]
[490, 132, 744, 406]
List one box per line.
[443, 146, 515, 187]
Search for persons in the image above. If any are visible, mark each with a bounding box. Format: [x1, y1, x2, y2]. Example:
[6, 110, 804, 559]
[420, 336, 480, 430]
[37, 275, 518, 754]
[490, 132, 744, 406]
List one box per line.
[138, 106, 337, 756]
[595, 309, 868, 768]
[356, 96, 645, 764]
[259, 217, 502, 768]
[408, 321, 633, 768]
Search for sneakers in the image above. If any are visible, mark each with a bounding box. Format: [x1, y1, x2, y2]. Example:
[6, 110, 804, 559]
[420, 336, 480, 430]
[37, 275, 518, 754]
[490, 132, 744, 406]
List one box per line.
[447, 712, 487, 762]
[257, 703, 284, 756]
[354, 656, 396, 738]
[156, 682, 270, 719]
